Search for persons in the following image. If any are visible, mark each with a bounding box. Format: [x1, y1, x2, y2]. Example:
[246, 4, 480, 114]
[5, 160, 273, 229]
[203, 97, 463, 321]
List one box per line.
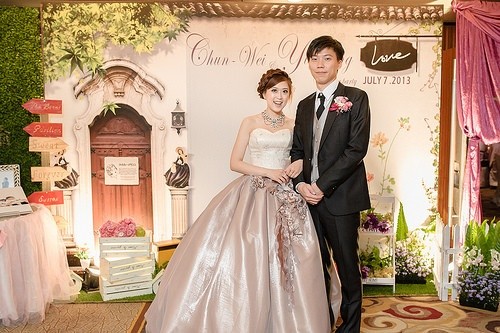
[2, 177, 9, 189]
[290, 35, 372, 333]
[143, 68, 341, 333]
[0, 195, 29, 207]
[163, 147, 190, 188]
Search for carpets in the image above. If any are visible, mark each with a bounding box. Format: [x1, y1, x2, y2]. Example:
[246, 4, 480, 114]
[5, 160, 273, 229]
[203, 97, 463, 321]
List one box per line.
[0, 293, 500, 333]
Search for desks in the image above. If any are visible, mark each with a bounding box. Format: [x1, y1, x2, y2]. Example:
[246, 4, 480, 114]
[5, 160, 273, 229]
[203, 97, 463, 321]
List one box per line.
[0, 203, 71, 328]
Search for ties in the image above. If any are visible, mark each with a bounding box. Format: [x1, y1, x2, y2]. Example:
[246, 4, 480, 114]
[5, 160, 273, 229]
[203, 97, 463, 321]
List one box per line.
[316, 93, 326, 121]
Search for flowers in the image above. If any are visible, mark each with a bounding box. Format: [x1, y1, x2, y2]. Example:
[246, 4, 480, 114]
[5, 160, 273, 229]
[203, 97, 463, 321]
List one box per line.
[454, 217, 500, 311]
[97, 216, 139, 237]
[360, 240, 393, 278]
[328, 94, 345, 119]
[396, 230, 434, 282]
[361, 209, 393, 233]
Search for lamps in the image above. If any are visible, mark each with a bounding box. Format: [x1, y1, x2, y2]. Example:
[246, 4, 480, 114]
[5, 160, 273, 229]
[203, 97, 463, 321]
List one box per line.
[169, 99, 188, 134]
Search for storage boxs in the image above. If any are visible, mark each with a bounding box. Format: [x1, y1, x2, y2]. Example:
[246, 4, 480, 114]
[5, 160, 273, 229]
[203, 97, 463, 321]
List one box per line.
[98, 228, 158, 303]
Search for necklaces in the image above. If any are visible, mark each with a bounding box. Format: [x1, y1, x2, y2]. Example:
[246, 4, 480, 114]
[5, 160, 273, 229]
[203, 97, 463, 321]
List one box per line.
[261, 110, 285, 130]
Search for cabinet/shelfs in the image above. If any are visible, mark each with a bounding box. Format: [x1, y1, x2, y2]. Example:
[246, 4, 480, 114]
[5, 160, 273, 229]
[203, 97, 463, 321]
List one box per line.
[356, 194, 402, 295]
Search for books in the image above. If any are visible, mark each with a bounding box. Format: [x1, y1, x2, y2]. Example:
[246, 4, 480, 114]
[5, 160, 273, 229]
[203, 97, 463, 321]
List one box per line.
[0, 186, 32, 217]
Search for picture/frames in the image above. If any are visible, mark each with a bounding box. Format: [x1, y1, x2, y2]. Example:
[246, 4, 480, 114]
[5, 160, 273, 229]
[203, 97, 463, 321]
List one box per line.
[0, 164, 21, 188]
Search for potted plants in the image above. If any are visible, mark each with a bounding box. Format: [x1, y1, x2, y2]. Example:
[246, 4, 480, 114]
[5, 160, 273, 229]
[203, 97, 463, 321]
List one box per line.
[74, 242, 93, 270]
